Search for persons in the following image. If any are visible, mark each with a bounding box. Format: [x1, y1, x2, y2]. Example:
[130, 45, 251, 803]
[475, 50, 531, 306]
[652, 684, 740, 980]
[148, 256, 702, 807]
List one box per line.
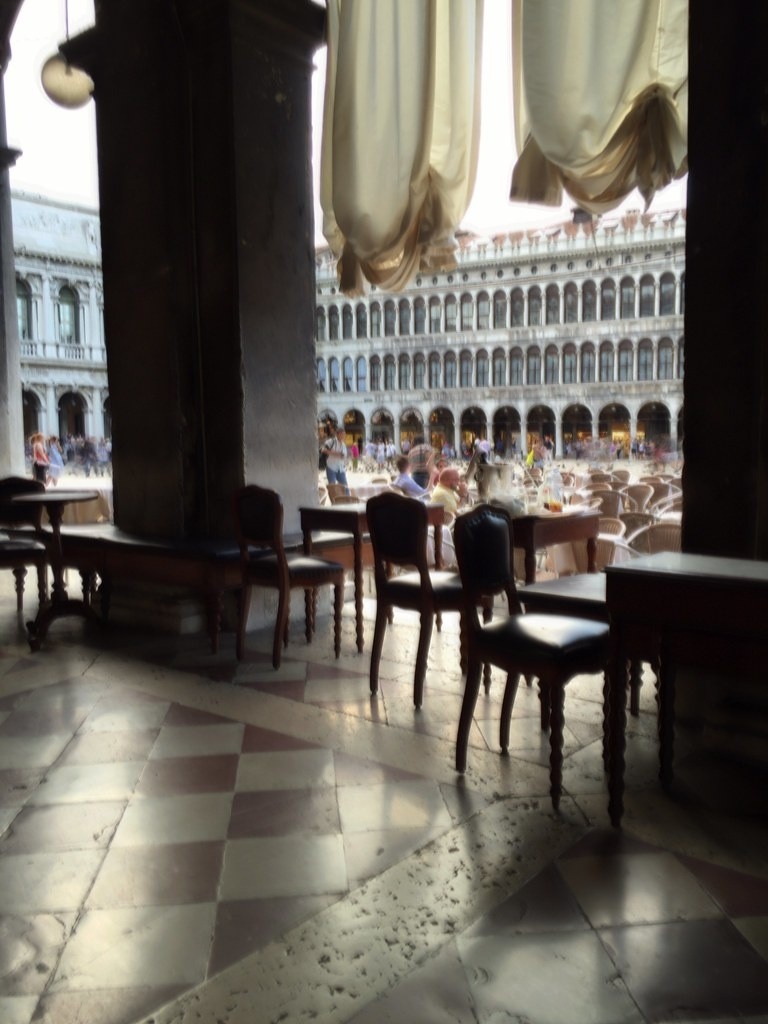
[320, 428, 350, 496]
[392, 457, 436, 497]
[352, 436, 685, 489]
[430, 468, 473, 517]
[25, 432, 112, 487]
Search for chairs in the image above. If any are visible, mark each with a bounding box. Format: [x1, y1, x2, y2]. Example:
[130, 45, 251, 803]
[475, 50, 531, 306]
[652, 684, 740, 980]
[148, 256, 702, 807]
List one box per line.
[365, 492, 492, 710]
[453, 503, 627, 830]
[46, 447, 683, 604]
[235, 483, 346, 671]
[0, 476, 49, 629]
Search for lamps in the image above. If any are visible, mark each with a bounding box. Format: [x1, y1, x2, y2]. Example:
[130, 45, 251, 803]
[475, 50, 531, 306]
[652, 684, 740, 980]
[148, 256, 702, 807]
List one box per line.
[40, 0, 94, 108]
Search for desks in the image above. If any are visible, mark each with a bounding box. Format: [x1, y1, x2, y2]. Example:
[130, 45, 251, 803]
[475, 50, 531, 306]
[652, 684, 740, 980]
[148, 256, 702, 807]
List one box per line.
[12, 491, 99, 654]
[602, 551, 768, 831]
[510, 510, 599, 690]
[298, 498, 445, 661]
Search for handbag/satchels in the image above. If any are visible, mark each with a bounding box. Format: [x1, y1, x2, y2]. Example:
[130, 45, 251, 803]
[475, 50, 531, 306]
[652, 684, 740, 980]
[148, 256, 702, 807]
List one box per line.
[318, 438, 334, 470]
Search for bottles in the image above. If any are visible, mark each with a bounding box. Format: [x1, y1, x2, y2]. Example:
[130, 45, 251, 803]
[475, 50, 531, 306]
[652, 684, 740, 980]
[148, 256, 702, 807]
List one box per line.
[548, 468, 564, 512]
